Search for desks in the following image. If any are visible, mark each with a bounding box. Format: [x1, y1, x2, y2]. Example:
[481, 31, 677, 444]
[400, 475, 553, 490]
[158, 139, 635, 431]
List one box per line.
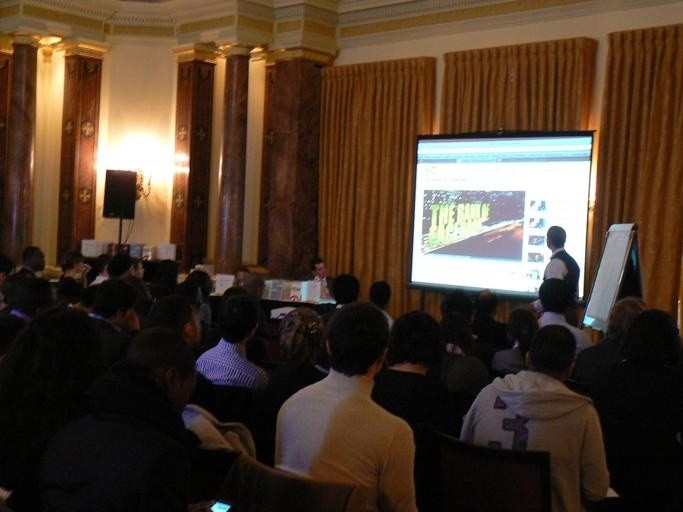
[79, 252, 180, 300]
[197, 290, 339, 359]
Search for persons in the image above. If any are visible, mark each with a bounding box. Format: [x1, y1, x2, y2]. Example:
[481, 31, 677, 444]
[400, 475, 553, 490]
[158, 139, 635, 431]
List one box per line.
[273, 301, 419, 512]
[458, 323, 610, 512]
[526, 226, 581, 328]
[1, 244, 683, 511]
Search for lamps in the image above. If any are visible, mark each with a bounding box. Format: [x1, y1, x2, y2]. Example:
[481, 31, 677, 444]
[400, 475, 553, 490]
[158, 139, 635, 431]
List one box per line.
[129, 168, 153, 200]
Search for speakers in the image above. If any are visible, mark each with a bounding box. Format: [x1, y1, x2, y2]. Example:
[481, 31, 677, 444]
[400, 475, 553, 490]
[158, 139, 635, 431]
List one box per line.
[103, 169, 137, 220]
